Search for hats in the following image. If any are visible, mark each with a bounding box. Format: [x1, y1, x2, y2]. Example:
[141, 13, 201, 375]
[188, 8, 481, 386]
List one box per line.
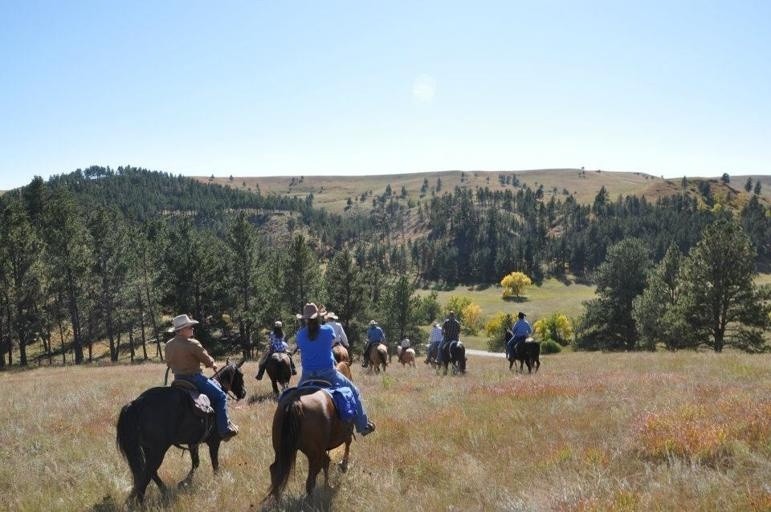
[295, 302, 328, 320]
[166, 313, 201, 333]
[324, 311, 340, 320]
[369, 320, 378, 325]
[275, 321, 283, 328]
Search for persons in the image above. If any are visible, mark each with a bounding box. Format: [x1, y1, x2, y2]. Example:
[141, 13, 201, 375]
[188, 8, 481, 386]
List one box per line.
[434, 311, 460, 365]
[295, 302, 375, 436]
[165, 314, 239, 442]
[361, 320, 385, 367]
[424, 321, 443, 364]
[507, 311, 532, 361]
[322, 312, 354, 364]
[397, 335, 410, 362]
[255, 320, 297, 379]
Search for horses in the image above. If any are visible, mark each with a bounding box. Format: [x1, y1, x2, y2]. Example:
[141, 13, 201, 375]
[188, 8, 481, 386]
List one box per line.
[503, 325, 541, 376]
[114, 354, 247, 506]
[333, 343, 349, 364]
[425, 340, 466, 376]
[259, 358, 354, 505]
[365, 340, 389, 375]
[264, 344, 292, 397]
[395, 343, 416, 369]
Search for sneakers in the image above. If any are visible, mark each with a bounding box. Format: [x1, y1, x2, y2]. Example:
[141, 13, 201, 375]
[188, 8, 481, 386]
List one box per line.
[218, 423, 240, 443]
[362, 421, 376, 436]
